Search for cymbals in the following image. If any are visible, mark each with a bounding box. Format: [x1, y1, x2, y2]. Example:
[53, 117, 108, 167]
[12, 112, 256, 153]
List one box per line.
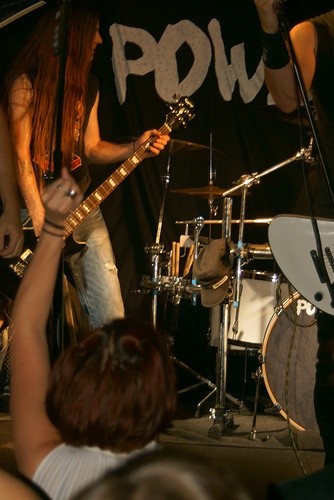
[169, 183, 252, 196]
[130, 135, 215, 155]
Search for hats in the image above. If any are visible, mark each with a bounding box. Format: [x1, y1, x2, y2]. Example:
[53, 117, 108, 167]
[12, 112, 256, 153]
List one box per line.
[193, 239, 237, 308]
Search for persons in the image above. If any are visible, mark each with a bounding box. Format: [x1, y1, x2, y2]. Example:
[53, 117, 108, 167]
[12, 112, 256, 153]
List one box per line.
[0, 80, 23, 260]
[255, 0, 334, 471]
[0, 168, 273, 500]
[8, 0, 170, 328]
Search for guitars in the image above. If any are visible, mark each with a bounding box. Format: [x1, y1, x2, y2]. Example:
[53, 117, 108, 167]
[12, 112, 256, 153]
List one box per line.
[1, 91, 198, 332]
[266, 213, 333, 316]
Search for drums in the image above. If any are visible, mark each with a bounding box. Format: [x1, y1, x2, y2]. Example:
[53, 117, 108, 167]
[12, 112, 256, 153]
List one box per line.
[205, 242, 298, 355]
[260, 291, 334, 434]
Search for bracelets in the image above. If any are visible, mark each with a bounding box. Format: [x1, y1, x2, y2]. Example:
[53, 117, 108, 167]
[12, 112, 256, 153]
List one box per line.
[132, 141, 136, 152]
[41, 227, 65, 238]
[44, 217, 65, 230]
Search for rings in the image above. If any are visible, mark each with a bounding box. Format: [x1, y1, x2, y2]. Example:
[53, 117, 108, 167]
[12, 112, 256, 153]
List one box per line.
[57, 184, 64, 190]
[66, 190, 77, 197]
